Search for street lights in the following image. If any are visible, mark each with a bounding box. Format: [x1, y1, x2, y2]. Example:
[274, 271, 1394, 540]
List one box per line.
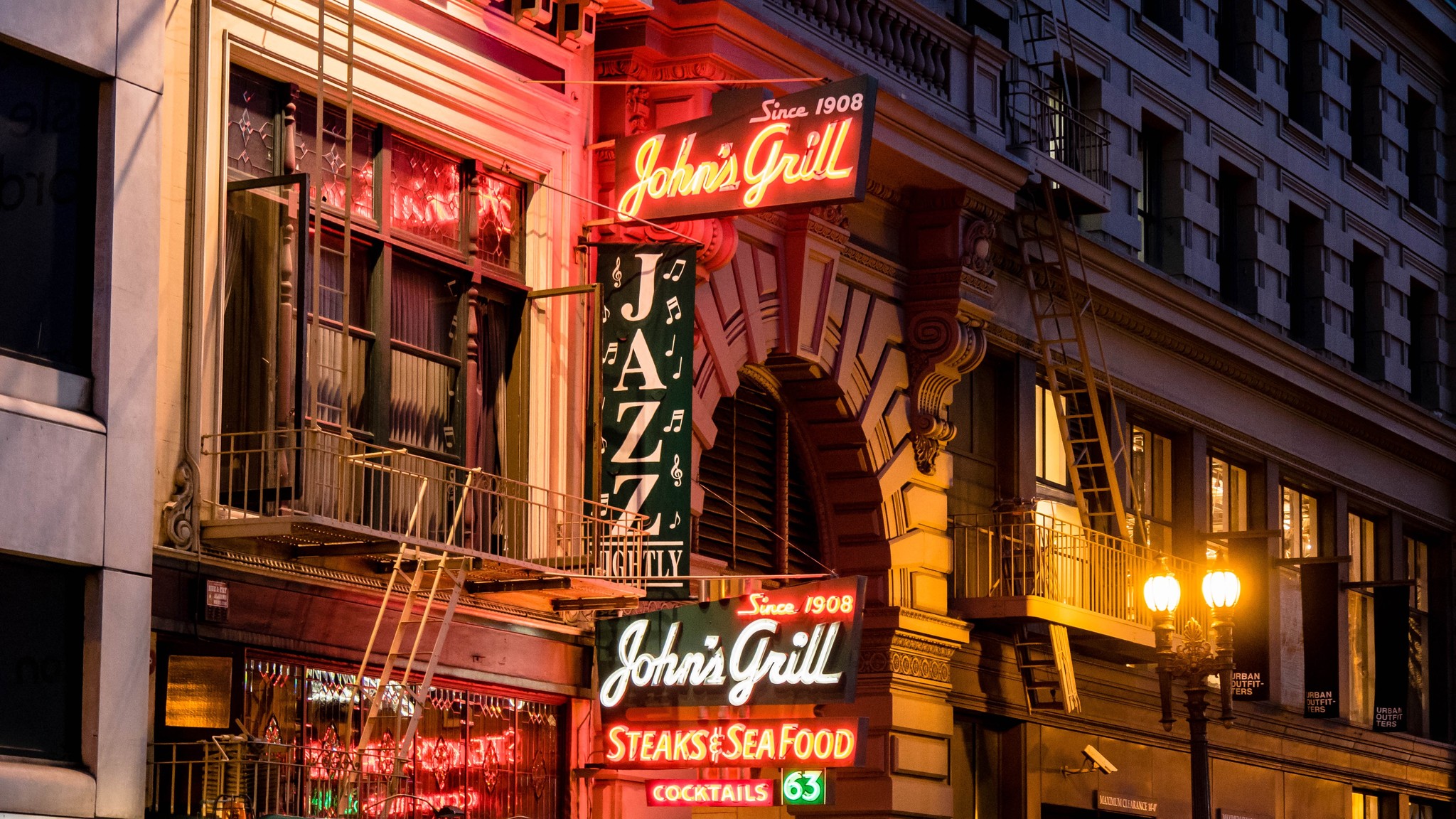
[1142, 570, 1241, 819]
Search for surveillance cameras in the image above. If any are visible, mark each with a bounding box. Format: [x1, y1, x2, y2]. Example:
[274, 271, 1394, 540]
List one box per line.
[1081, 744, 1119, 774]
